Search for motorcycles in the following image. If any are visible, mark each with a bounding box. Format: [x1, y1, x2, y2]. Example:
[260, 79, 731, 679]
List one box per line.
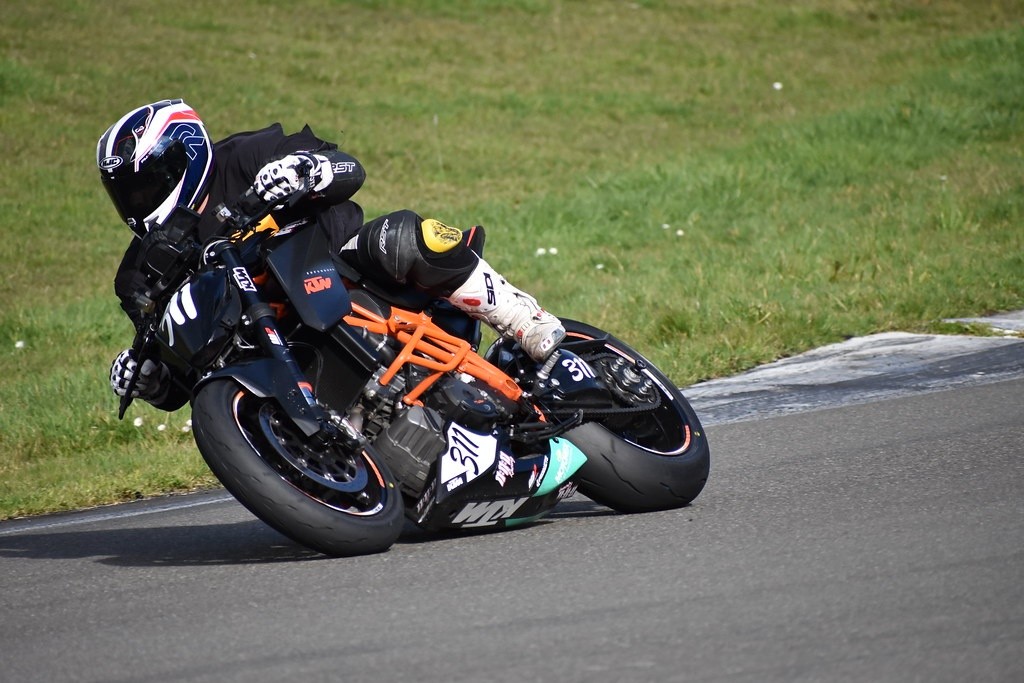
[118, 159, 712, 558]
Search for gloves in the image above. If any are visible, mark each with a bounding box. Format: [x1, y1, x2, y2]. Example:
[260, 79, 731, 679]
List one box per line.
[252, 151, 309, 201]
[108, 349, 170, 404]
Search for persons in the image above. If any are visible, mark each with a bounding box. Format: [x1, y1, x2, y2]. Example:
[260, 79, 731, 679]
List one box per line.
[96, 98, 567, 413]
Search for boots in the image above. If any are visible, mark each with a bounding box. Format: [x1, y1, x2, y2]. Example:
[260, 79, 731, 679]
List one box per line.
[440, 250, 566, 360]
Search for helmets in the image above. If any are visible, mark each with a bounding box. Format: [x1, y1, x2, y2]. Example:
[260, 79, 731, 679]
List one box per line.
[95, 99, 218, 241]
